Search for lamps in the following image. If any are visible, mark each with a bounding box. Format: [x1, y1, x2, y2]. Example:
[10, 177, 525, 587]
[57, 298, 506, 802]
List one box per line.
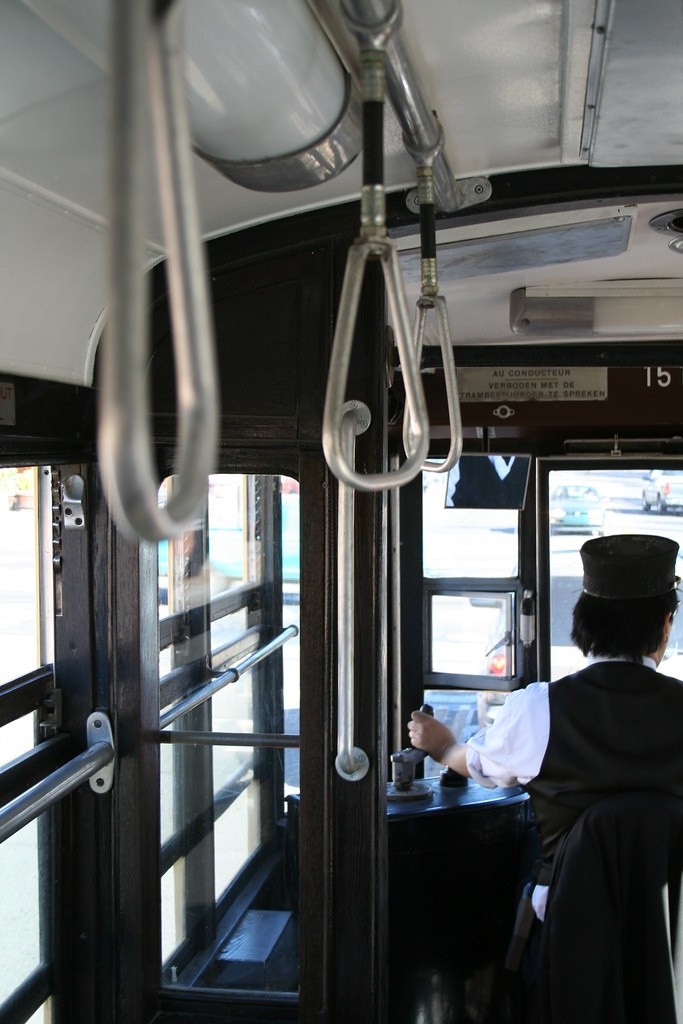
[20, 0, 363, 193]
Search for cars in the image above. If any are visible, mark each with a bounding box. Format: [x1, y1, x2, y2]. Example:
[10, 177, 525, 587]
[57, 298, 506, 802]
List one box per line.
[469, 538, 683, 683]
[549, 482, 611, 538]
[141, 471, 299, 613]
[640, 469, 683, 515]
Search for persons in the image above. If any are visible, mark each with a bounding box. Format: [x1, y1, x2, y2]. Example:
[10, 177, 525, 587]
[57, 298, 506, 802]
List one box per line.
[407, 534, 683, 1024]
[451, 455, 530, 509]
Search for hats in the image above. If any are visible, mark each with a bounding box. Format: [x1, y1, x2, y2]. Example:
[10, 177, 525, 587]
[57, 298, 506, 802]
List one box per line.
[580, 534, 680, 598]
